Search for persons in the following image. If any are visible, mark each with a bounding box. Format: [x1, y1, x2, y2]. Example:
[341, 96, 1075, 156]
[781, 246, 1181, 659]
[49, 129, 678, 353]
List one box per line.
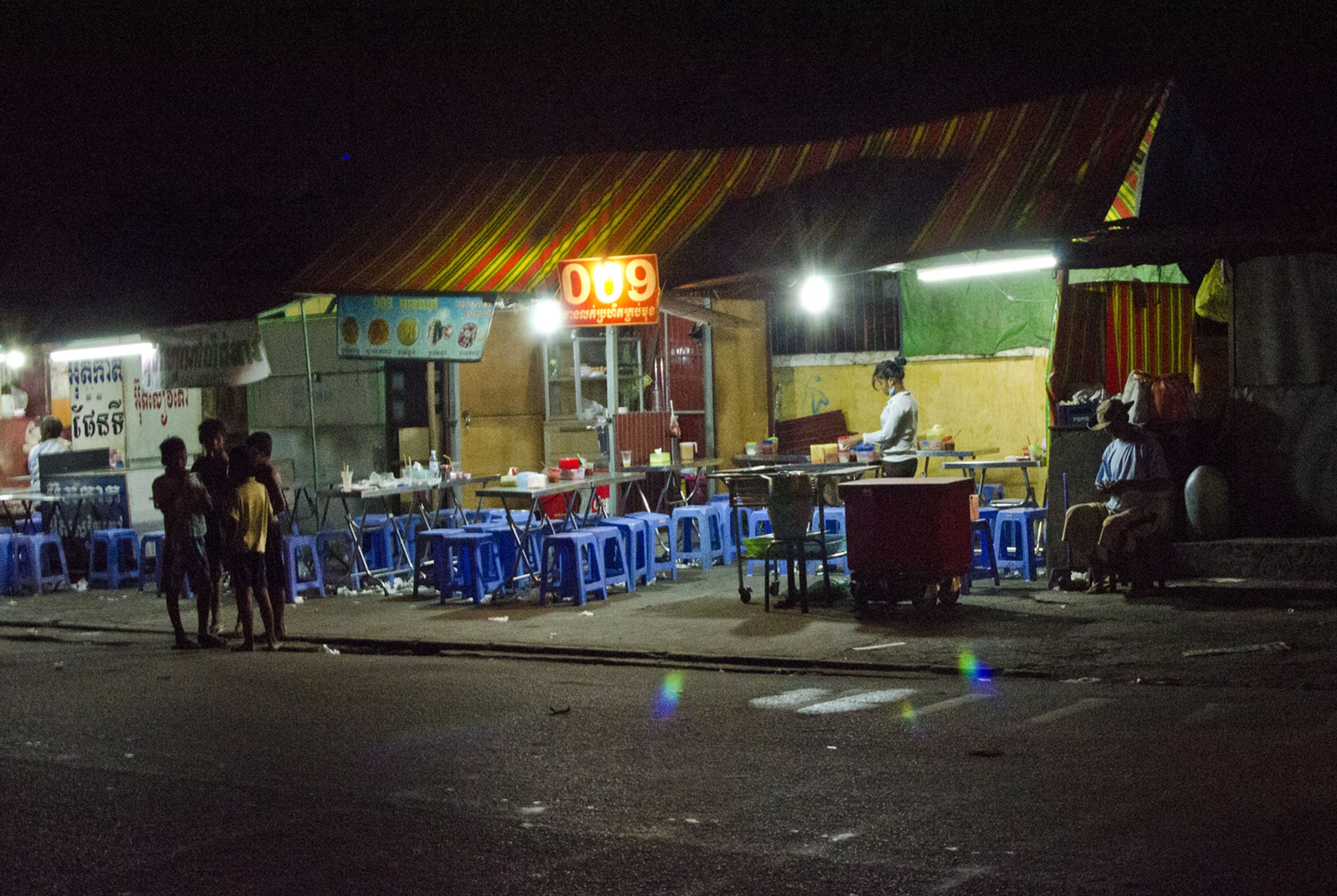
[152, 417, 286, 651]
[28, 415, 73, 511]
[1062, 399, 1171, 597]
[842, 356, 916, 479]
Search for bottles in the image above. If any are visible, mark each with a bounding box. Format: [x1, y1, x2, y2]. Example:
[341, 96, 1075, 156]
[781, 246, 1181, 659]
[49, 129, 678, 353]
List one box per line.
[429, 450, 438, 478]
[1023, 438, 1047, 460]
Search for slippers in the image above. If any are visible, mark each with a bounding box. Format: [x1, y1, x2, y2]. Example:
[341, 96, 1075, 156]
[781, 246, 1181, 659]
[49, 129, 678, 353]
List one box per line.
[1088, 581, 1099, 593]
[1103, 574, 1118, 592]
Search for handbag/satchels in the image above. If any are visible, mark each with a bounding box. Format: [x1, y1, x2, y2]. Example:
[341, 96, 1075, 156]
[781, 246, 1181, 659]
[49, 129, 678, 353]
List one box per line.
[1150, 373, 1197, 421]
[1122, 370, 1159, 423]
[1195, 259, 1233, 322]
[670, 400, 681, 436]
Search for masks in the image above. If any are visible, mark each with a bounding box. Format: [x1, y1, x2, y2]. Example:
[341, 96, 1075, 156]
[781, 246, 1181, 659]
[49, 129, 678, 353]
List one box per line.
[888, 380, 894, 396]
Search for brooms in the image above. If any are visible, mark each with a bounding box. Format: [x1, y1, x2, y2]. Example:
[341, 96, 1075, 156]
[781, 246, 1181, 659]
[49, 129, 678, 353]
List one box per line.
[1048, 473, 1089, 591]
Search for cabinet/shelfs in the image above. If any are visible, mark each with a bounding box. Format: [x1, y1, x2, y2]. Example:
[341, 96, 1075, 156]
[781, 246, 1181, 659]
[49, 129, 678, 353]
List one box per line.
[540, 327, 643, 422]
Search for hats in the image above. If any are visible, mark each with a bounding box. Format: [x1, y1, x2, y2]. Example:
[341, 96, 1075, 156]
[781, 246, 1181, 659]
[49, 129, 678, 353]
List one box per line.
[1087, 399, 1135, 431]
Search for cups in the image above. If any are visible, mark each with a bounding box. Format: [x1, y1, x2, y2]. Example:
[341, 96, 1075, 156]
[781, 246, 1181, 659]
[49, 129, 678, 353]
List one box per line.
[945, 438, 955, 451]
[809, 435, 882, 464]
[341, 471, 354, 492]
[621, 450, 632, 467]
[401, 461, 462, 486]
[583, 462, 594, 477]
[746, 436, 779, 456]
[184, 471, 201, 488]
[548, 467, 561, 483]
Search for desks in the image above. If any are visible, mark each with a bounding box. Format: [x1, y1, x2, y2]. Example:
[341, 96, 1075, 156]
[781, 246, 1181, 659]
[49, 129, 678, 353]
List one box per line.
[836, 476, 976, 605]
[324, 474, 501, 592]
[918, 446, 1001, 477]
[616, 456, 728, 563]
[0, 489, 108, 574]
[477, 470, 672, 600]
[945, 460, 1047, 510]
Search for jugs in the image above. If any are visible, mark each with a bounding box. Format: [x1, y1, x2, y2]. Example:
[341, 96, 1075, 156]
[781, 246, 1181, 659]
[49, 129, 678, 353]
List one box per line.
[679, 442, 698, 460]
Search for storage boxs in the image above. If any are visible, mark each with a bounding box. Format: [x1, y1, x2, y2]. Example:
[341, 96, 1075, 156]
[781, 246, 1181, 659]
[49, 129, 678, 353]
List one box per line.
[1057, 402, 1100, 426]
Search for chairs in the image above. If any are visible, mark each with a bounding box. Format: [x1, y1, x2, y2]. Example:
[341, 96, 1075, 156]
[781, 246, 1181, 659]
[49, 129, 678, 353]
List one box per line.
[1086, 502, 1174, 593]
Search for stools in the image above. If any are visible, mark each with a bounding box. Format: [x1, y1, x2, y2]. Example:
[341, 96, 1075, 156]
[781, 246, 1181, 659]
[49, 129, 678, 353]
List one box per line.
[0, 493, 1049, 609]
